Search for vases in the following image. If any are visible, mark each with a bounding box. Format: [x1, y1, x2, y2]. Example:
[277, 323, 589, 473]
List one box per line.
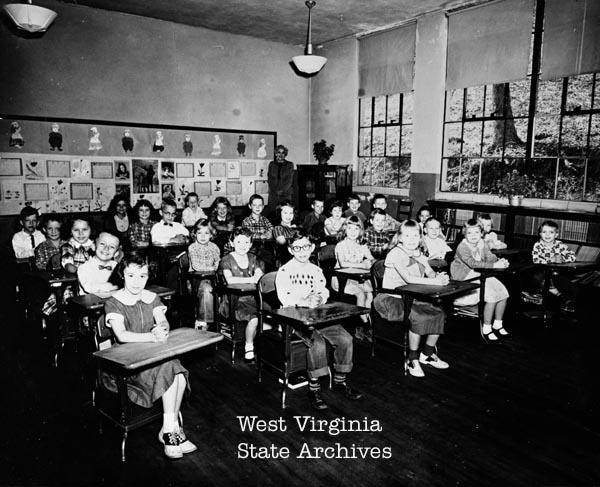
[318, 158, 329, 165]
[509, 195, 524, 206]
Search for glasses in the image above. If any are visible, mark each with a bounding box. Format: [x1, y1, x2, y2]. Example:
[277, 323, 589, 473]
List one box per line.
[292, 245, 310, 252]
[165, 212, 176, 218]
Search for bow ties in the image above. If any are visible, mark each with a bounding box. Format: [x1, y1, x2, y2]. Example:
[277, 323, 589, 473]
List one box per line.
[164, 222, 173, 226]
[99, 265, 113, 270]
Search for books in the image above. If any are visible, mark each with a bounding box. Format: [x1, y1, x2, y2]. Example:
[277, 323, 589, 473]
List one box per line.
[515, 215, 600, 288]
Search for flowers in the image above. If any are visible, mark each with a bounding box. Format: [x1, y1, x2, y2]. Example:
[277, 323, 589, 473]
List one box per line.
[488, 170, 537, 198]
[312, 139, 335, 159]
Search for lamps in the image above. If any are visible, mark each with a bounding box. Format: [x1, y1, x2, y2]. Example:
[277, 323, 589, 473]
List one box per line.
[4, 0, 58, 33]
[292, 1, 327, 74]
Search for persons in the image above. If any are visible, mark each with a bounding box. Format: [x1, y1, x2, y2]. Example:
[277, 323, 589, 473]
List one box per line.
[526, 220, 576, 312]
[373, 219, 450, 378]
[217, 227, 272, 364]
[275, 231, 363, 410]
[450, 219, 514, 343]
[104, 256, 198, 459]
[12, 146, 508, 326]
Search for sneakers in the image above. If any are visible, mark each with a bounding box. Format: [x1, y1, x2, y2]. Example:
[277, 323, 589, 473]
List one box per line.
[491, 327, 512, 341]
[195, 320, 207, 330]
[245, 342, 255, 359]
[331, 379, 363, 399]
[419, 352, 450, 369]
[405, 358, 426, 377]
[483, 331, 503, 345]
[310, 388, 327, 410]
[159, 426, 197, 459]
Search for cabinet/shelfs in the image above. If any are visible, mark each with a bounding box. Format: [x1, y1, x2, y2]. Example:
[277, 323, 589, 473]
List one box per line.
[295, 162, 354, 215]
[426, 197, 598, 249]
